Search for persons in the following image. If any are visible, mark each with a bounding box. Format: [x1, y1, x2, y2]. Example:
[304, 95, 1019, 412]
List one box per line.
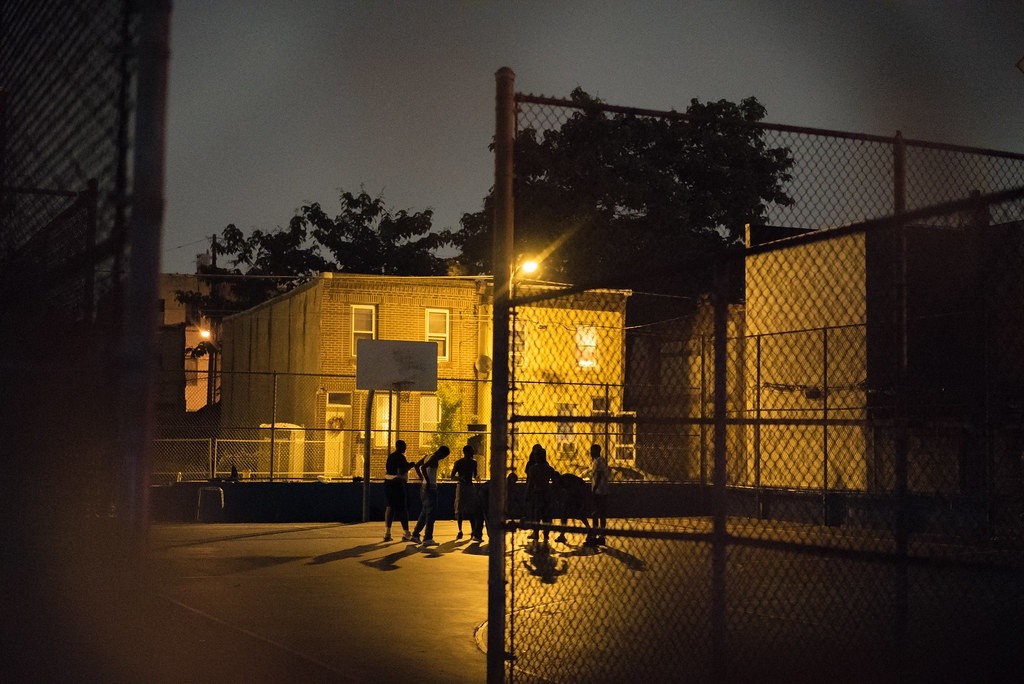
[451, 446, 518, 541]
[384, 440, 415, 540]
[524, 444, 608, 543]
[410, 446, 450, 546]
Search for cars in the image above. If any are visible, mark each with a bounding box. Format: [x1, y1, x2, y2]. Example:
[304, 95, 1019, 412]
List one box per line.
[577, 462, 670, 482]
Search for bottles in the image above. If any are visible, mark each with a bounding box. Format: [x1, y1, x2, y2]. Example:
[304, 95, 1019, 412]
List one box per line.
[177, 472, 182, 482]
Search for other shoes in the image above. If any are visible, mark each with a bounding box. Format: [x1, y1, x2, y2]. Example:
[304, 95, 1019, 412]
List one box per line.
[423, 539, 440, 546]
[473, 536, 484, 544]
[457, 531, 462, 538]
[402, 536, 411, 541]
[598, 537, 605, 545]
[527, 533, 539, 539]
[384, 534, 394, 541]
[555, 537, 566, 543]
[409, 535, 422, 544]
[583, 538, 598, 546]
